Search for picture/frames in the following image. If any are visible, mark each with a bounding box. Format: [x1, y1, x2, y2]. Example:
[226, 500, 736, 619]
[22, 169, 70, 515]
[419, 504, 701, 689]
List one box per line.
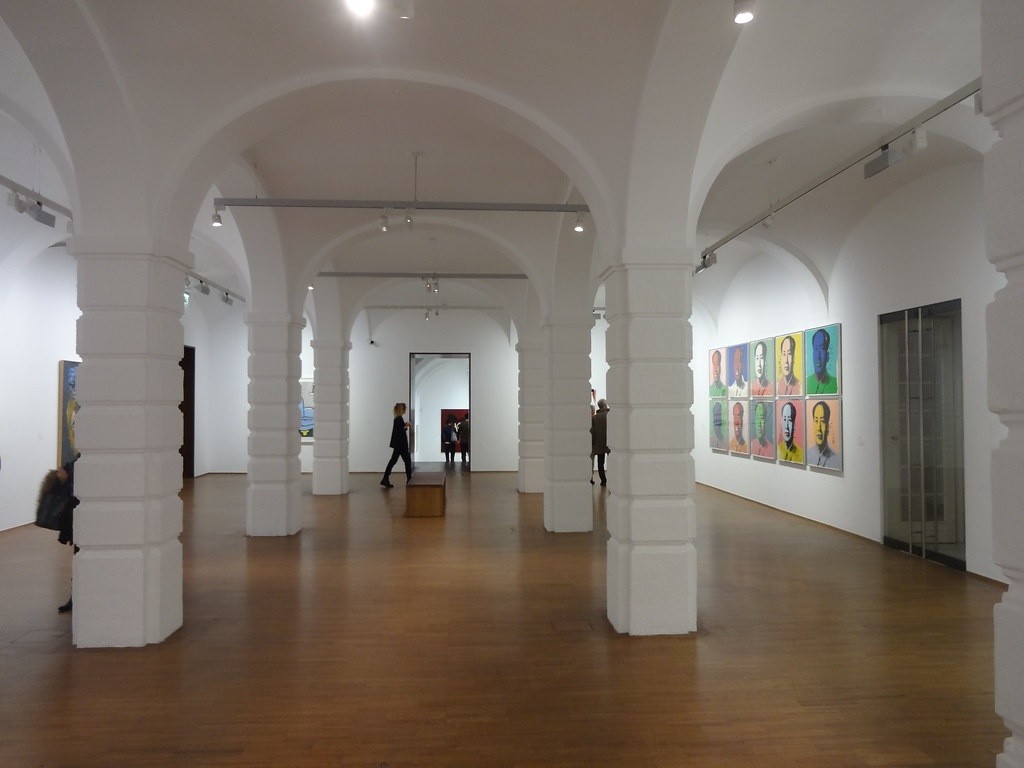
[708, 321, 844, 472]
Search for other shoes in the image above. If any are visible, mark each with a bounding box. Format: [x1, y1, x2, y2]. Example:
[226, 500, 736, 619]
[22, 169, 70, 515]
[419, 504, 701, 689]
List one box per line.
[58, 597, 72, 611]
[600, 480, 606, 486]
[381, 477, 394, 488]
[590, 481, 595, 484]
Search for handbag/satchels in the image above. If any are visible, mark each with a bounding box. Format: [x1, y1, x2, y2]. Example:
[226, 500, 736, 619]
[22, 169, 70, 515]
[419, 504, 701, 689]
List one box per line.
[451, 432, 458, 441]
[33, 479, 69, 530]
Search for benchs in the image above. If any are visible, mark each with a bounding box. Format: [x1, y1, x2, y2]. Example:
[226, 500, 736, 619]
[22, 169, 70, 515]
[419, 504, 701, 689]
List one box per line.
[405, 472, 447, 518]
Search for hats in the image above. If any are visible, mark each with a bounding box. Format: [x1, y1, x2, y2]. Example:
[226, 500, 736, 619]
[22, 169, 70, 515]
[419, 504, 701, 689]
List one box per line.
[597, 399, 606, 407]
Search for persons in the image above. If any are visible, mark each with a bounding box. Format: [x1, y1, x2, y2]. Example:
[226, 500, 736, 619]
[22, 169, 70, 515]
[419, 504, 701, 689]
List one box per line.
[443, 412, 471, 464]
[54, 454, 77, 614]
[590, 398, 611, 487]
[380, 402, 413, 488]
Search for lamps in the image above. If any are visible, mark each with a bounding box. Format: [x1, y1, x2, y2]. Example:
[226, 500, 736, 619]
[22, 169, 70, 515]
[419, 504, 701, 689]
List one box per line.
[183, 273, 191, 289]
[196, 281, 210, 296]
[435, 308, 440, 317]
[423, 276, 430, 293]
[210, 204, 226, 225]
[12, 195, 57, 228]
[423, 308, 430, 321]
[432, 276, 439, 294]
[218, 291, 233, 305]
[695, 252, 716, 274]
[376, 206, 389, 233]
[760, 213, 774, 229]
[732, 1, 757, 25]
[401, 207, 413, 223]
[571, 210, 586, 231]
[307, 279, 315, 293]
[865, 143, 903, 180]
